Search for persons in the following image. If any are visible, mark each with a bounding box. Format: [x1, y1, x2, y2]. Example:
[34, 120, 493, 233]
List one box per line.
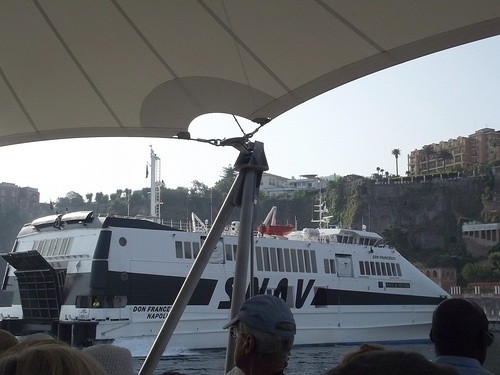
[93, 298, 100, 307]
[257, 222, 266, 237]
[222, 294, 296, 375]
[0, 329, 108, 375]
[323, 297, 495, 375]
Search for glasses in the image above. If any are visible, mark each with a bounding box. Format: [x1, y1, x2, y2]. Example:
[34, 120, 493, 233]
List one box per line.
[484, 330, 494, 346]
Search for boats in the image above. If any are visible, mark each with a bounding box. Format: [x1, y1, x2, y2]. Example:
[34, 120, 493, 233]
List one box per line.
[0, 145, 457, 356]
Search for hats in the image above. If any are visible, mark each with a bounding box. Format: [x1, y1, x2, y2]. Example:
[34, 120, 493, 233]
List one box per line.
[84, 343, 138, 375]
[222, 294, 296, 337]
